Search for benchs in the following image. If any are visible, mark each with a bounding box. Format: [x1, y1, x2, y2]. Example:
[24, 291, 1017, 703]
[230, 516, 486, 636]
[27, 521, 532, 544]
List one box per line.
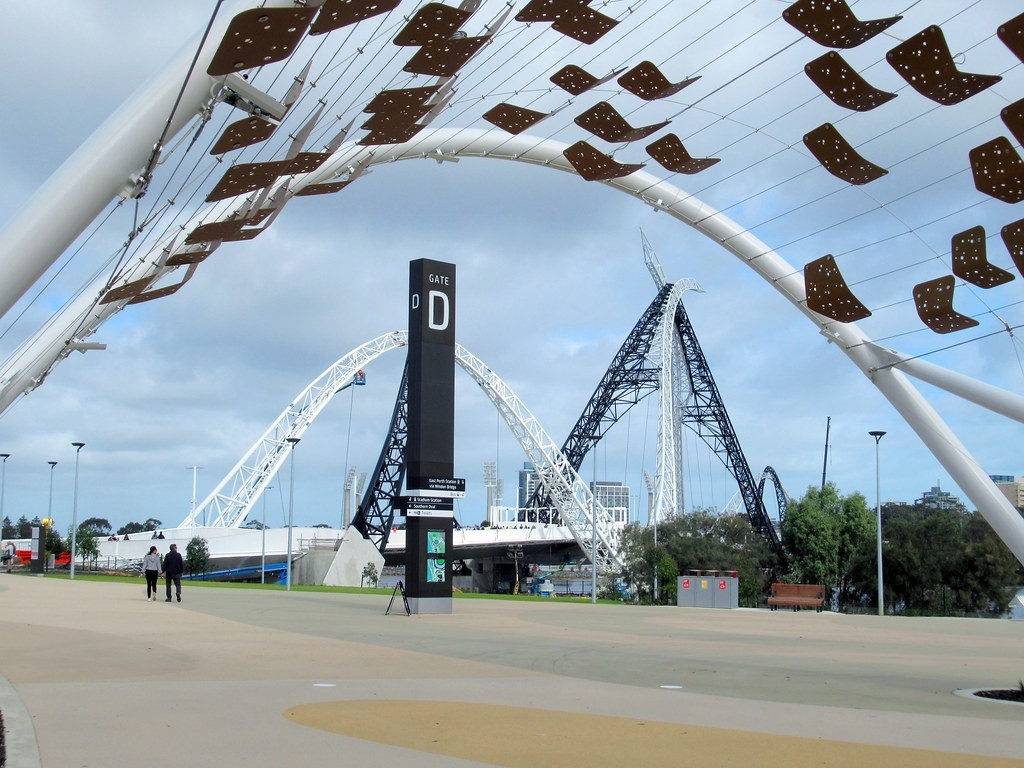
[767, 584, 827, 612]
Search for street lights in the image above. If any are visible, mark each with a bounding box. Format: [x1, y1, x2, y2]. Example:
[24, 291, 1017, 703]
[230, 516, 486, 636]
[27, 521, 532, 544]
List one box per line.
[70, 442, 86, 578]
[285, 437, 302, 592]
[186, 466, 206, 540]
[587, 435, 603, 603]
[261, 486, 275, 584]
[868, 431, 887, 616]
[0, 454, 10, 539]
[48, 461, 59, 518]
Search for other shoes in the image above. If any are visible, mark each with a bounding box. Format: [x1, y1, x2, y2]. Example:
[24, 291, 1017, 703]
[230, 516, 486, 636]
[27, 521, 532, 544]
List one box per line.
[148, 598, 152, 601]
[153, 592, 156, 601]
[165, 598, 171, 602]
[176, 592, 181, 602]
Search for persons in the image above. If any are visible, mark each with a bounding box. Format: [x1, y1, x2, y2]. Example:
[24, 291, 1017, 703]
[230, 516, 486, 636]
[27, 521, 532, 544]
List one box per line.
[109, 534, 118, 541]
[162, 544, 184, 602]
[141, 547, 162, 601]
[124, 533, 129, 539]
[153, 531, 165, 540]
